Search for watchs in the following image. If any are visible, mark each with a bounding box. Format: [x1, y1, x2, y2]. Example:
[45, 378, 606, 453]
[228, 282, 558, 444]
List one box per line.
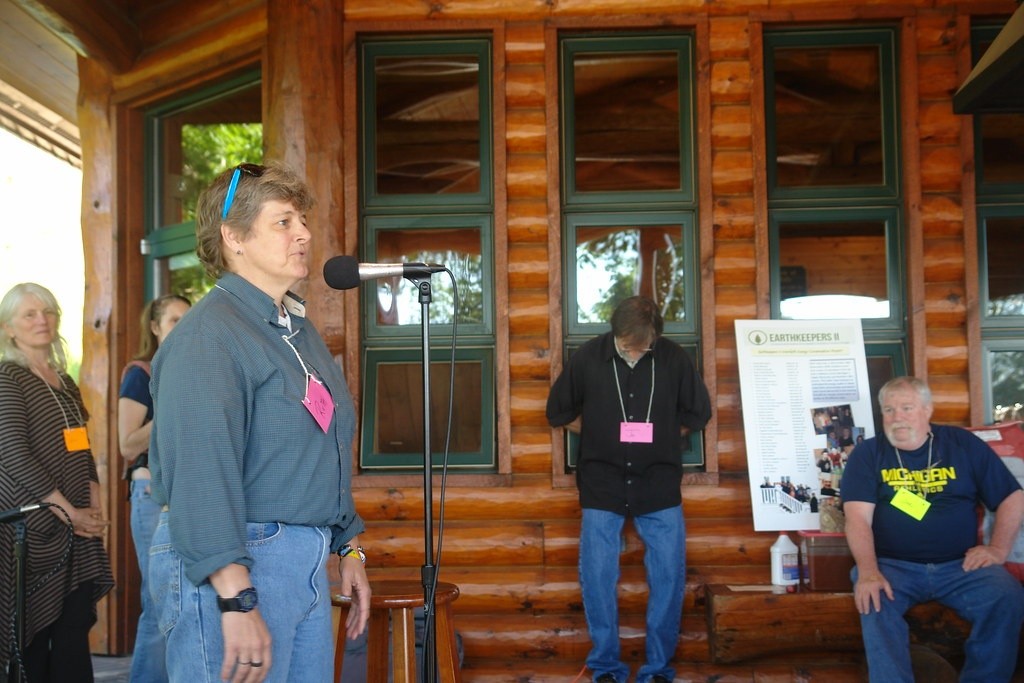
[217, 587, 259, 613]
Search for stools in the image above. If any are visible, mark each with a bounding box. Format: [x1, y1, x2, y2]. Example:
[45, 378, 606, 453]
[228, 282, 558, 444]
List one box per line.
[330, 580, 460, 683]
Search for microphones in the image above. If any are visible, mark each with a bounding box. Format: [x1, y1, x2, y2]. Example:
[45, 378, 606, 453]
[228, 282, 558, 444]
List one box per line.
[323, 256, 446, 289]
[0, 502, 51, 523]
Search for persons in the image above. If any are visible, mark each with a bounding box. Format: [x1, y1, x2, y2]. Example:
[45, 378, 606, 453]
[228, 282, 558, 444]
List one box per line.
[149, 160, 372, 683]
[117, 295, 193, 683]
[760, 405, 864, 514]
[0, 283, 114, 683]
[841, 375, 1024, 683]
[544, 296, 714, 683]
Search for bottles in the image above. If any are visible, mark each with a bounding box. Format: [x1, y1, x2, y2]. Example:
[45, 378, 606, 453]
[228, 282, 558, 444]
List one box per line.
[801, 536, 810, 585]
[770, 531, 800, 585]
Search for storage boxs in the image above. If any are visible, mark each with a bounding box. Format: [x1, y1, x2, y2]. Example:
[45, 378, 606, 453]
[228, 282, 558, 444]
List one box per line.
[797, 531, 854, 590]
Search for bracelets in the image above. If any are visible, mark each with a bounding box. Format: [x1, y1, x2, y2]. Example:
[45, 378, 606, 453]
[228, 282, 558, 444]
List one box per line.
[337, 544, 367, 568]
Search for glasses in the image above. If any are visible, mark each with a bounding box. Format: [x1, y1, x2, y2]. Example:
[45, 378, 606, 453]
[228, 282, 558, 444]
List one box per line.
[621, 348, 654, 353]
[220, 163, 264, 222]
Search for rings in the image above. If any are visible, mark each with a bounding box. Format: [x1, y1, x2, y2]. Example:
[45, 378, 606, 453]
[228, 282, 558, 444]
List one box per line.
[249, 661, 263, 668]
[237, 661, 251, 665]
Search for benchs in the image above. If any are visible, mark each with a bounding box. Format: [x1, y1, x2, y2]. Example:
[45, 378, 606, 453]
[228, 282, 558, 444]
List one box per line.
[705, 582, 978, 663]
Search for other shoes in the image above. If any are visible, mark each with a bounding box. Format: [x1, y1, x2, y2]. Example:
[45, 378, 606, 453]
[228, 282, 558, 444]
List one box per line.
[597, 675, 616, 683]
[648, 675, 669, 683]
